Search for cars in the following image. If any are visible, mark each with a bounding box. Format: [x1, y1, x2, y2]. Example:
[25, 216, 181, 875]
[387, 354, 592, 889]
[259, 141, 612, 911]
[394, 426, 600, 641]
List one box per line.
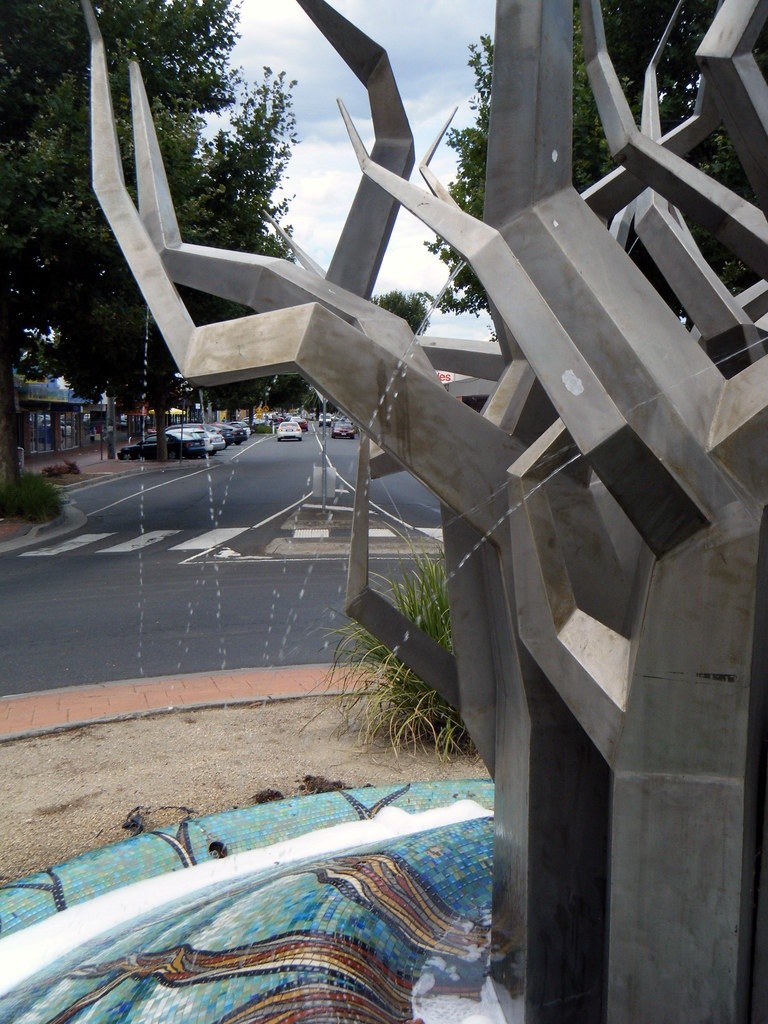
[164, 427, 215, 453]
[275, 421, 303, 442]
[289, 417, 302, 422]
[231, 407, 300, 436]
[27, 410, 91, 438]
[330, 410, 360, 433]
[331, 421, 355, 440]
[211, 420, 248, 447]
[117, 431, 207, 461]
[164, 423, 226, 456]
[297, 419, 309, 432]
[115, 411, 154, 430]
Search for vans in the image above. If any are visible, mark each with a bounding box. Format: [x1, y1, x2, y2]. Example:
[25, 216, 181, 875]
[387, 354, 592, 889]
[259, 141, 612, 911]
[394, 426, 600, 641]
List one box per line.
[318, 413, 332, 427]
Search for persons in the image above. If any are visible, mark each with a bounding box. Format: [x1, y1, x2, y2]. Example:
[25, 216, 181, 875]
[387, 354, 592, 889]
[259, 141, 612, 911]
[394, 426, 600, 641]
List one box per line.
[222, 417, 226, 422]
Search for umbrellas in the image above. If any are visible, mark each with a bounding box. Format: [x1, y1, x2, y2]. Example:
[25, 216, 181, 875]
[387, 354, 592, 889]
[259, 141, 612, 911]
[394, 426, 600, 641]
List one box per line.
[148, 408, 188, 427]
